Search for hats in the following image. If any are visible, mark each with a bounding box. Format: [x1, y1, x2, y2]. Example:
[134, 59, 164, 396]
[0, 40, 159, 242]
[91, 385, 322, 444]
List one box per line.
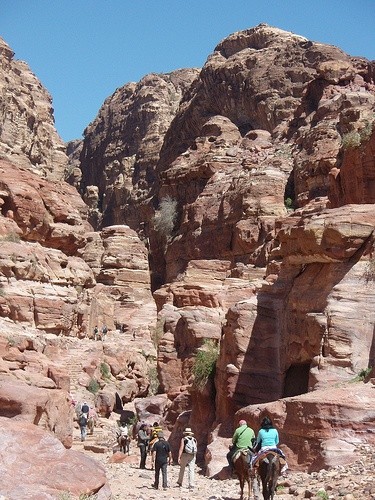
[239, 420, 247, 424]
[183, 428, 194, 436]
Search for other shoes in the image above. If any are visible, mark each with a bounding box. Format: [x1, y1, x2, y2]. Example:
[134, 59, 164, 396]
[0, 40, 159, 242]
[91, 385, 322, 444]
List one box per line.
[162, 485, 169, 490]
[152, 483, 159, 488]
[174, 482, 182, 488]
[189, 483, 198, 488]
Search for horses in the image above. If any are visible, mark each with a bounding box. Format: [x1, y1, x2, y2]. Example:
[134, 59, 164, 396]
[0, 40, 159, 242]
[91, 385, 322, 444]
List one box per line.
[116, 431, 131, 456]
[226, 444, 288, 500]
[78, 417, 96, 434]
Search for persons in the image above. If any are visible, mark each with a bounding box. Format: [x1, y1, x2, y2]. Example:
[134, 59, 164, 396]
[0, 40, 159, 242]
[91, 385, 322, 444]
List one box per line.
[151, 432, 171, 489]
[78, 402, 97, 442]
[253, 416, 280, 452]
[148, 422, 163, 445]
[138, 424, 151, 469]
[227, 420, 255, 472]
[177, 427, 197, 489]
[120, 422, 129, 436]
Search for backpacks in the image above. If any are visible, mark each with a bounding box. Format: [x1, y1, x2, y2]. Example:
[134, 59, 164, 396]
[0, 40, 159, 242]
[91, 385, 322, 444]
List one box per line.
[184, 436, 198, 454]
[78, 416, 87, 426]
